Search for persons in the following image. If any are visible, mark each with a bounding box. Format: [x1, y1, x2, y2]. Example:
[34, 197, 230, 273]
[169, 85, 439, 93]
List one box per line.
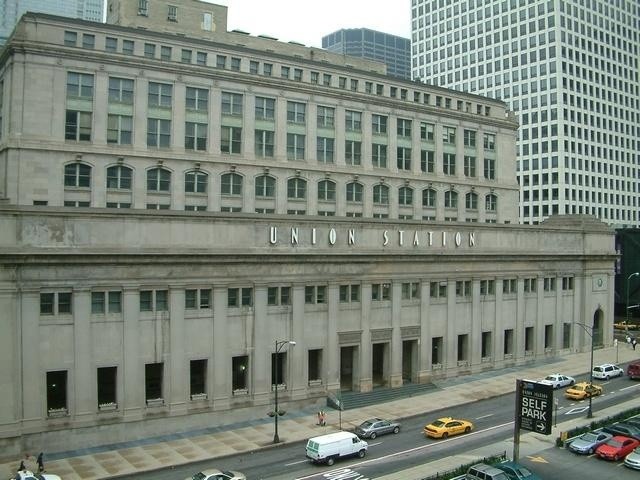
[625, 333, 637, 350]
[36, 452, 44, 472]
[20, 460, 25, 470]
[317, 410, 327, 426]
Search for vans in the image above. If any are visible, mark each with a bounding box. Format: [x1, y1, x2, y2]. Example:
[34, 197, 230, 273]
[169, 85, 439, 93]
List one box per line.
[592, 363, 624, 381]
[465, 461, 511, 480]
[627, 359, 640, 380]
[305, 430, 369, 467]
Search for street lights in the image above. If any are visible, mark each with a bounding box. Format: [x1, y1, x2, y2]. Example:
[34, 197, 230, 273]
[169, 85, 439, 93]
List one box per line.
[267, 339, 297, 444]
[625, 272, 639, 342]
[567, 320, 595, 418]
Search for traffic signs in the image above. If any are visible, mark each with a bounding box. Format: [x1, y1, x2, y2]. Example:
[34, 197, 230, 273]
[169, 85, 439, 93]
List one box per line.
[520, 381, 551, 435]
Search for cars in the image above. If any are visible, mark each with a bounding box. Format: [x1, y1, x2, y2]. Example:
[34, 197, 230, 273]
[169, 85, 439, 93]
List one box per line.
[594, 435, 640, 462]
[354, 417, 402, 440]
[492, 461, 540, 480]
[564, 381, 603, 400]
[568, 430, 613, 455]
[602, 423, 640, 442]
[185, 467, 247, 480]
[9, 469, 63, 480]
[538, 373, 576, 390]
[423, 416, 473, 440]
[614, 320, 639, 330]
[623, 446, 640, 470]
